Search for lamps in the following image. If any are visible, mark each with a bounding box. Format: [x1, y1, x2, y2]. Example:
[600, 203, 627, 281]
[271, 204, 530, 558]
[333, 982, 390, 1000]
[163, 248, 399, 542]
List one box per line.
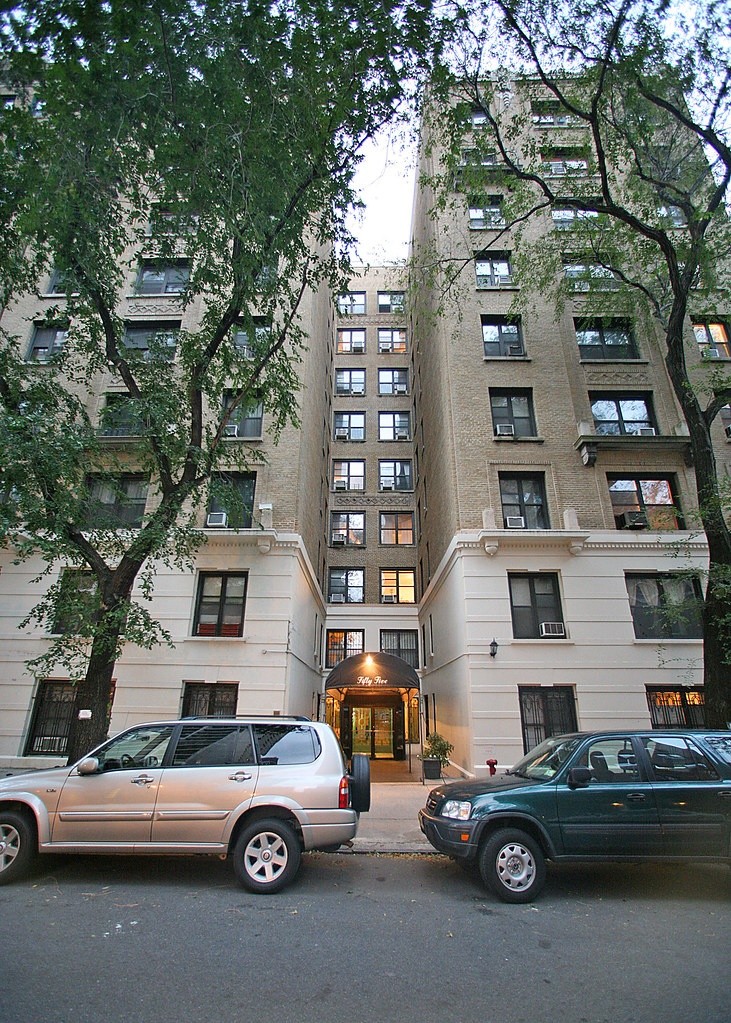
[489, 638, 498, 658]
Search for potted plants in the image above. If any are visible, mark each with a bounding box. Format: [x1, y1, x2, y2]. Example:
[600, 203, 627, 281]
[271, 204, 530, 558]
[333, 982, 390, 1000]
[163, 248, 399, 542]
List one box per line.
[416, 731, 454, 779]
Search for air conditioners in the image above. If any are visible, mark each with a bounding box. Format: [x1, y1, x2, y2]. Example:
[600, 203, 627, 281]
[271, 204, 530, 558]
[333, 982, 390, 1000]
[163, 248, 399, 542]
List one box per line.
[332, 305, 411, 545]
[620, 510, 648, 530]
[497, 275, 514, 286]
[37, 737, 61, 752]
[225, 424, 240, 437]
[701, 348, 719, 358]
[505, 515, 525, 529]
[230, 345, 250, 358]
[495, 424, 514, 436]
[207, 512, 229, 528]
[539, 621, 565, 638]
[506, 345, 524, 356]
[383, 594, 395, 604]
[637, 428, 656, 436]
[551, 166, 568, 175]
[332, 594, 343, 603]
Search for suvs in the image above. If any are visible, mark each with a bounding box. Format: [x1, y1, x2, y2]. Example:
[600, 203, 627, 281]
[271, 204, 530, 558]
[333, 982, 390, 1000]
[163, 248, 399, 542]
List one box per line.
[417, 731, 731, 905]
[0, 712, 371, 895]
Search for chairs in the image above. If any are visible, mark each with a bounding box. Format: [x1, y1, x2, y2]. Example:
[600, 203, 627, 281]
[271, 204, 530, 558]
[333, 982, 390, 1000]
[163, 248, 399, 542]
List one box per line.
[612, 749, 641, 782]
[653, 749, 679, 781]
[589, 751, 614, 782]
[200, 744, 226, 764]
